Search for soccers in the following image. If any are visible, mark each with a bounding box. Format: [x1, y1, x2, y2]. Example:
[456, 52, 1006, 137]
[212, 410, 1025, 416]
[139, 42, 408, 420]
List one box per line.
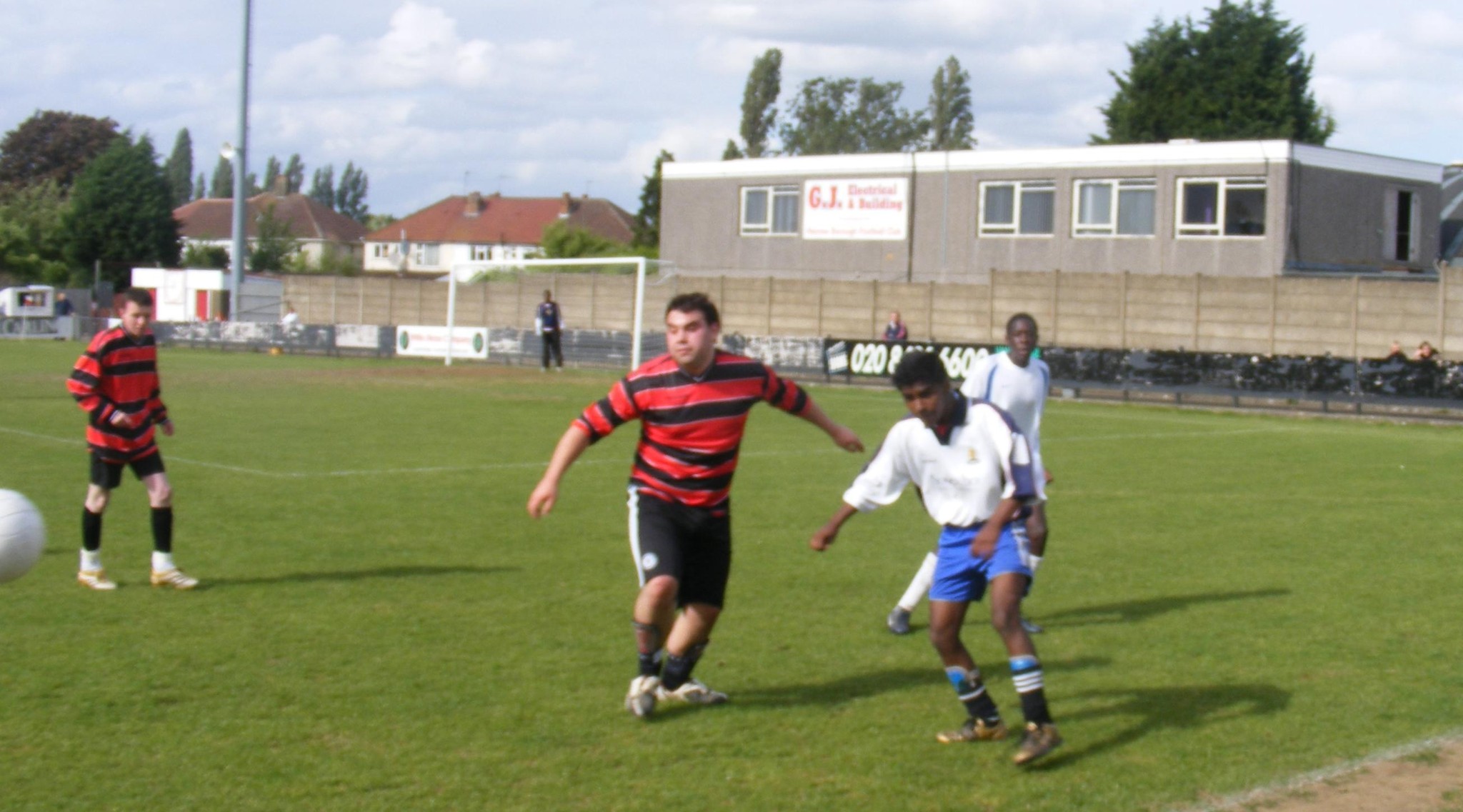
[0, 486, 49, 586]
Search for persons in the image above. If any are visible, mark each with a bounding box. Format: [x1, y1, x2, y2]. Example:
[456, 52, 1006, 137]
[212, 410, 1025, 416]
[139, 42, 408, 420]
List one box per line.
[1410, 341, 1442, 360]
[885, 311, 909, 340]
[279, 308, 298, 323]
[54, 292, 76, 318]
[886, 312, 1053, 635]
[1386, 339, 1408, 360]
[528, 292, 867, 720]
[805, 351, 1064, 765]
[536, 290, 565, 373]
[66, 288, 198, 590]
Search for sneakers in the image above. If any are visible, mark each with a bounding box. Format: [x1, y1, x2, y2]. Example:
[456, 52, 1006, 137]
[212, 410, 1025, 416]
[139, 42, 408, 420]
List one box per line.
[77, 569, 117, 591]
[658, 681, 728, 705]
[935, 718, 1007, 745]
[625, 677, 654, 719]
[150, 567, 199, 590]
[887, 608, 912, 634]
[1022, 620, 1043, 634]
[1013, 722, 1061, 762]
